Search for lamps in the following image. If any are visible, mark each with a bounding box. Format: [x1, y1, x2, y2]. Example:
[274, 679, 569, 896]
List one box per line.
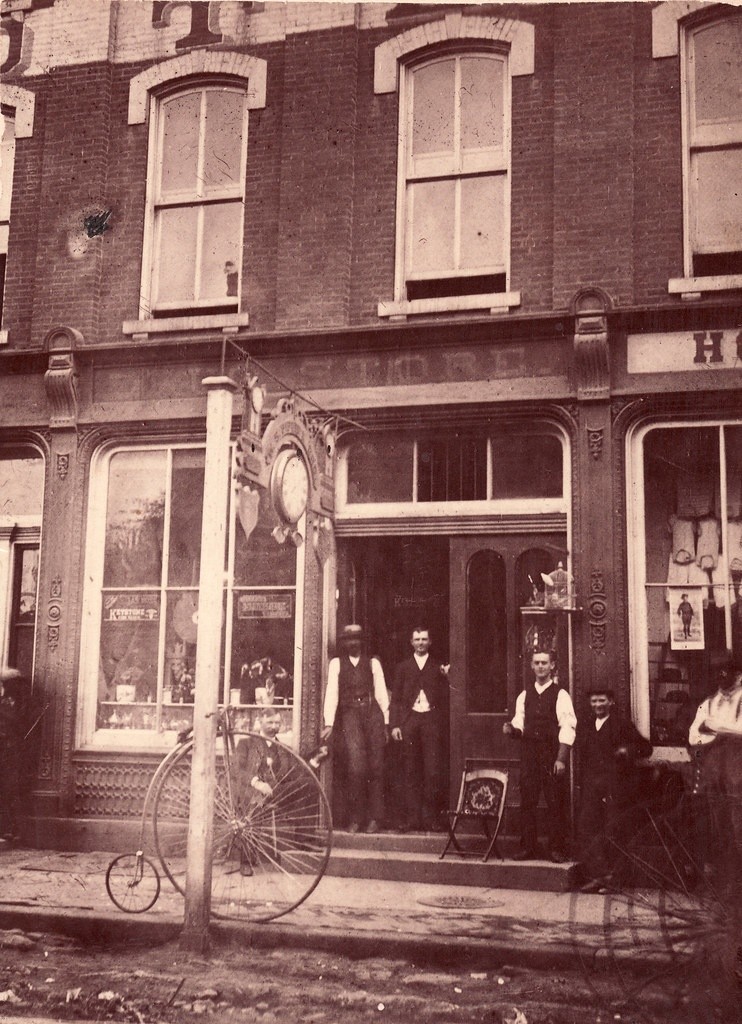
[86, 210, 114, 237]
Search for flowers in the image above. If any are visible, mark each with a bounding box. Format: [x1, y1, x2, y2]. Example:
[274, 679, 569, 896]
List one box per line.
[240, 655, 289, 696]
[115, 665, 142, 686]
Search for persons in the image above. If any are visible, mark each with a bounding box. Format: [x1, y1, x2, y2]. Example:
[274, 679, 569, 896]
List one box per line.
[229, 708, 327, 875]
[688, 651, 742, 854]
[321, 625, 391, 834]
[583, 686, 652, 893]
[678, 594, 694, 640]
[389, 628, 450, 833]
[501, 649, 578, 861]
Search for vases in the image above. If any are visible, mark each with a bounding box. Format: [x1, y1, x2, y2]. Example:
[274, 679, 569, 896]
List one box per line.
[255, 688, 282, 704]
[115, 683, 137, 703]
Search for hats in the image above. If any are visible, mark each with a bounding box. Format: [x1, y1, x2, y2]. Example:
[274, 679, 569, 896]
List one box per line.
[338, 625, 363, 638]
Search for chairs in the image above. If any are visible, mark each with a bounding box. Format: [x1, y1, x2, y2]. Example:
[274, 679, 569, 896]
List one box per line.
[438, 756, 514, 863]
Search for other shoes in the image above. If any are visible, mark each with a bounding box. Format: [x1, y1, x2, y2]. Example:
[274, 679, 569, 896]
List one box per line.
[512, 850, 530, 859]
[398, 822, 416, 830]
[431, 823, 442, 832]
[366, 819, 378, 834]
[348, 822, 360, 834]
[582, 874, 622, 894]
